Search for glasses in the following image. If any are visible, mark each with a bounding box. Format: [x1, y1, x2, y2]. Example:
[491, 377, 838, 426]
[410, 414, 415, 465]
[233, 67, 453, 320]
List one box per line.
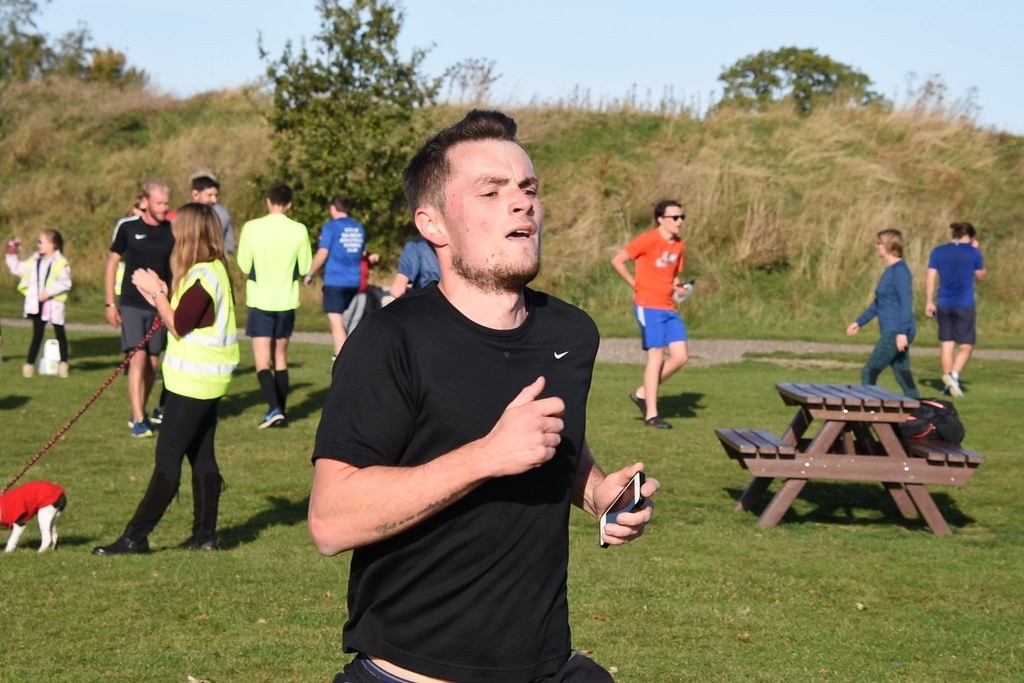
[661, 215, 685, 221]
[135, 205, 146, 213]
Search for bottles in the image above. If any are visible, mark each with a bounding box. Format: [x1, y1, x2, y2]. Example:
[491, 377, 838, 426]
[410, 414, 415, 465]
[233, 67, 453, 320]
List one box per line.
[39, 339, 61, 375]
[672, 279, 696, 303]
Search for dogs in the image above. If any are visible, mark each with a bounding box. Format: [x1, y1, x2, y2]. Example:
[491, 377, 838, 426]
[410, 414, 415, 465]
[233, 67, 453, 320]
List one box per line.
[0, 481, 67, 552]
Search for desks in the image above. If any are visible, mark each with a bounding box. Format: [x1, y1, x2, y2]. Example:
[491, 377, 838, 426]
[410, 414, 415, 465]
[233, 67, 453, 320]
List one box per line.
[736, 384, 951, 535]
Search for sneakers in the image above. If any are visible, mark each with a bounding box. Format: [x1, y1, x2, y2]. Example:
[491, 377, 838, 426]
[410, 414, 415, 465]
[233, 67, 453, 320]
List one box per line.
[630, 389, 647, 418]
[942, 370, 964, 399]
[645, 416, 671, 430]
[127, 414, 159, 438]
[257, 407, 288, 430]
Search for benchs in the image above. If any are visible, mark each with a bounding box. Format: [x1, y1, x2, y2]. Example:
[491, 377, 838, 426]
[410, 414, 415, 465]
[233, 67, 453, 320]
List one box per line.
[714, 428, 796, 456]
[909, 438, 985, 464]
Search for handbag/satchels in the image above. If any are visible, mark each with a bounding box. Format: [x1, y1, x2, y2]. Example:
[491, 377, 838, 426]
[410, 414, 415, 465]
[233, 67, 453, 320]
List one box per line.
[895, 398, 965, 444]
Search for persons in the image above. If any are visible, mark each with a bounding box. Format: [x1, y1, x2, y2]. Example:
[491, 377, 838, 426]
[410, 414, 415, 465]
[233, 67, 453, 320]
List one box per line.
[6, 229, 72, 378]
[392, 236, 440, 298]
[308, 111, 660, 682]
[304, 196, 365, 361]
[924, 222, 987, 397]
[191, 176, 235, 254]
[90, 202, 240, 557]
[237, 182, 312, 429]
[343, 251, 379, 335]
[847, 229, 921, 398]
[105, 180, 175, 438]
[611, 201, 688, 429]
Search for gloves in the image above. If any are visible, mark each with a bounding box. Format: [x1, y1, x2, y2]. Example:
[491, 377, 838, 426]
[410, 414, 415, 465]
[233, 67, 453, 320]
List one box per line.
[8, 241, 21, 254]
[39, 289, 49, 303]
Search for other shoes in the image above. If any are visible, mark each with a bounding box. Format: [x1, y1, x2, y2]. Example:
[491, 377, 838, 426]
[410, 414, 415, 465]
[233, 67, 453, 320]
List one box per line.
[150, 409, 165, 425]
[22, 363, 33, 378]
[58, 361, 69, 379]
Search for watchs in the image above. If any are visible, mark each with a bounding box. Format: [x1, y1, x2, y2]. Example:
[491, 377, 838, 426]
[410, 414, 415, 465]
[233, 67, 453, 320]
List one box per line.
[152, 291, 163, 299]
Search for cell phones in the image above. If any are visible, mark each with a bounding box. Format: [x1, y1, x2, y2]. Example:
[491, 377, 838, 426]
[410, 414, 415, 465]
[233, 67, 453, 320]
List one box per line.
[599, 471, 646, 548]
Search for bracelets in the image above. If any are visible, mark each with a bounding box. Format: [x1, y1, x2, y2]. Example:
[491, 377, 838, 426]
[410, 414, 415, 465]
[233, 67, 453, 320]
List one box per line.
[306, 275, 313, 277]
[105, 303, 116, 308]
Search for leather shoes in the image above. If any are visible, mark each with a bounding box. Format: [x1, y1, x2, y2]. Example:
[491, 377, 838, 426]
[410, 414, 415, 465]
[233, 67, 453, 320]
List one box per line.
[179, 536, 216, 552]
[93, 534, 152, 557]
